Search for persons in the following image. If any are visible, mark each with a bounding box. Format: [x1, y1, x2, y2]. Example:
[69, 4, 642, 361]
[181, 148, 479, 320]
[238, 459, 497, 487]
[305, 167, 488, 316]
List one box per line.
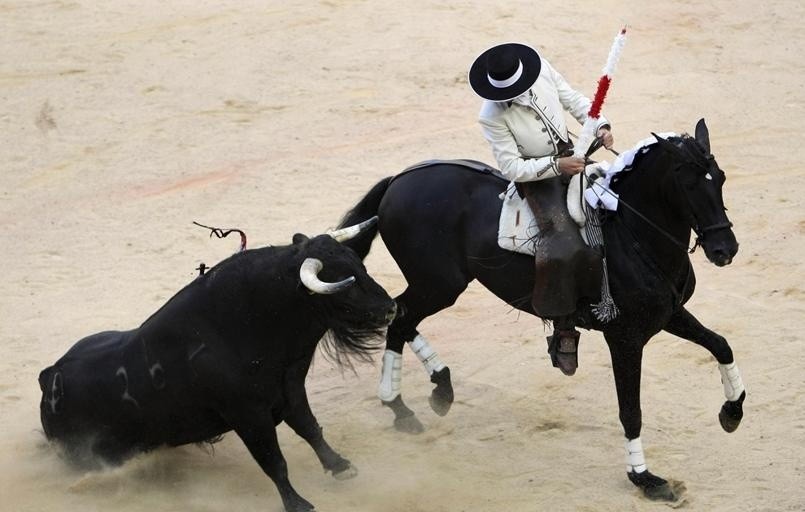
[470, 42, 614, 377]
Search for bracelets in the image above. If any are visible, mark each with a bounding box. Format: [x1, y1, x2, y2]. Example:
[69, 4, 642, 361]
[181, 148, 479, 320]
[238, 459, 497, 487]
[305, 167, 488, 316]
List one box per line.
[555, 158, 561, 173]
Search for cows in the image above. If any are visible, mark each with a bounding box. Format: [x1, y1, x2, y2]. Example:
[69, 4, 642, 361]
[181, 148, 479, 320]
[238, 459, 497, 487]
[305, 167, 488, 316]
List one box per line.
[39, 215, 380, 512]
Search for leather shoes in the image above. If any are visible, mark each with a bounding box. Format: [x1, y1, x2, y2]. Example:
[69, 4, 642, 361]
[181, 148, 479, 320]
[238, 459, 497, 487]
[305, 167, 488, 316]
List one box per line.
[558, 338, 576, 376]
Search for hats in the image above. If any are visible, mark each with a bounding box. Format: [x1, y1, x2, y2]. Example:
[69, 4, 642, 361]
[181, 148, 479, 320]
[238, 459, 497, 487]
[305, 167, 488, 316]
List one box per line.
[468, 42, 541, 103]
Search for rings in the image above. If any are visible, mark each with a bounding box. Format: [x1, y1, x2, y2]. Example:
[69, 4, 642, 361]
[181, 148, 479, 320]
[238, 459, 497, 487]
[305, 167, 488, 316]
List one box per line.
[576, 167, 579, 172]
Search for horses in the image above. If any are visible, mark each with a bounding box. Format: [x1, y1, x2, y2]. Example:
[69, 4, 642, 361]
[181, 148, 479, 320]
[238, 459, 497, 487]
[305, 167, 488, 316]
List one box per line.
[318, 118, 746, 507]
